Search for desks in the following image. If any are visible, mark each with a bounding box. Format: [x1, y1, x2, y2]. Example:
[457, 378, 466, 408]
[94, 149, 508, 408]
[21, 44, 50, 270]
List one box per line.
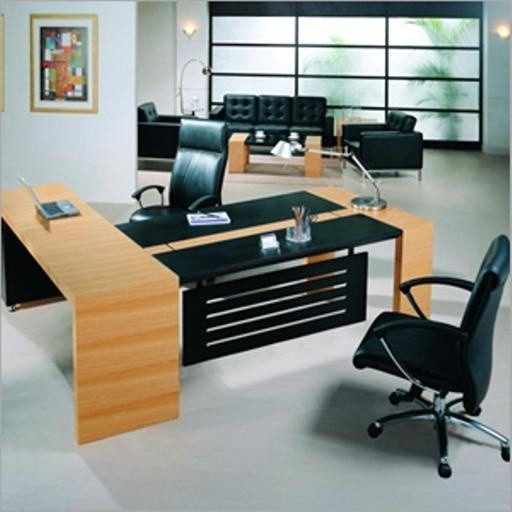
[117, 183, 435, 368]
[1, 182, 185, 444]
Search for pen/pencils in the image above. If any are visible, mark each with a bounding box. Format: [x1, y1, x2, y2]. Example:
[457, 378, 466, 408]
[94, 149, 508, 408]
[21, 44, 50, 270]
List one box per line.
[291, 206, 312, 240]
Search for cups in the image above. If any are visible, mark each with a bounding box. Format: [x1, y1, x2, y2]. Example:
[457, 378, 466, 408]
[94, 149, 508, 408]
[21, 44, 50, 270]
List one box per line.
[284, 220, 313, 243]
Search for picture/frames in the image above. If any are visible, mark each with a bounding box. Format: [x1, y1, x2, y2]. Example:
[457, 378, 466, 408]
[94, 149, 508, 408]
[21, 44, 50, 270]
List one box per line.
[27, 13, 97, 114]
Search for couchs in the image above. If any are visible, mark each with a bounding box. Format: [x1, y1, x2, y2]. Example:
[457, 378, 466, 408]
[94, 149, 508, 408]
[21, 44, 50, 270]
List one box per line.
[207, 95, 333, 154]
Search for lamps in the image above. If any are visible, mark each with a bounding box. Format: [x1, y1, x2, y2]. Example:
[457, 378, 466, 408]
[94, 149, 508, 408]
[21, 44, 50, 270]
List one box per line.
[177, 55, 211, 115]
[267, 136, 387, 210]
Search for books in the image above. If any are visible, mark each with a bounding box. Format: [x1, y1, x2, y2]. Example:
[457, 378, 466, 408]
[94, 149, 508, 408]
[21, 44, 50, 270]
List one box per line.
[186, 210, 230, 226]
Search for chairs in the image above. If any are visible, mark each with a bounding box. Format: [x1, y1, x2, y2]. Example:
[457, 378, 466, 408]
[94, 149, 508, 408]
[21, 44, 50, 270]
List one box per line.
[342, 110, 425, 185]
[128, 102, 227, 230]
[354, 233, 510, 480]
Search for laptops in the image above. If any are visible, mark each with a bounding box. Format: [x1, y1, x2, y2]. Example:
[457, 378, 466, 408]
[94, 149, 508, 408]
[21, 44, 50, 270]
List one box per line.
[17, 175, 80, 220]
